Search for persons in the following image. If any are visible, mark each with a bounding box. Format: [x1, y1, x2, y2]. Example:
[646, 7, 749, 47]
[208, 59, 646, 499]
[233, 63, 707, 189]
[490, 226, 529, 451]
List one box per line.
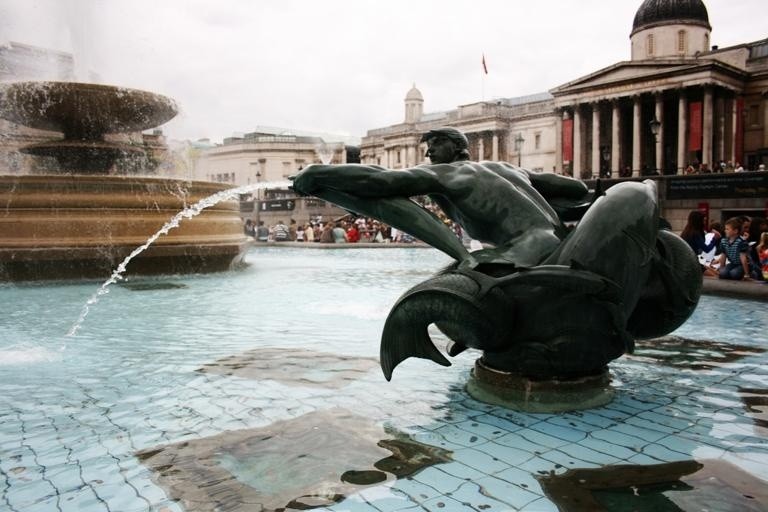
[681, 210, 768, 280]
[686, 157, 744, 174]
[244, 195, 464, 243]
[293, 127, 589, 265]
